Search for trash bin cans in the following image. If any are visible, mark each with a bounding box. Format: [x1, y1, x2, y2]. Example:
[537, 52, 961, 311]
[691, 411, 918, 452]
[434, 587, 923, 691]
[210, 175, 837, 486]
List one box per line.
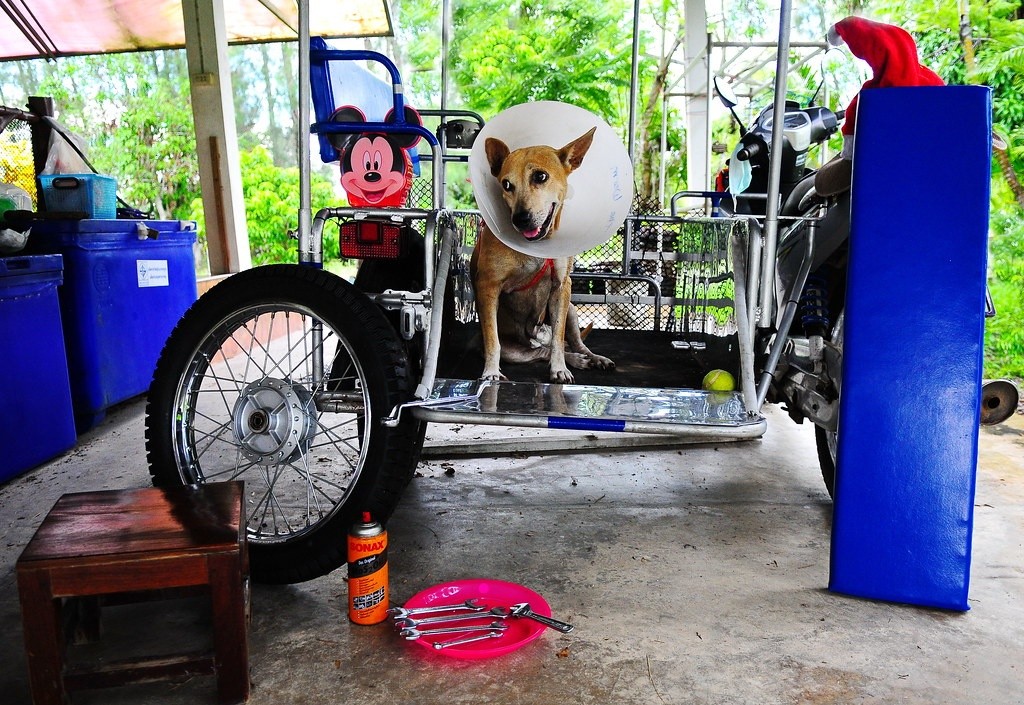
[79, 216, 202, 429]
[0, 252, 77, 484]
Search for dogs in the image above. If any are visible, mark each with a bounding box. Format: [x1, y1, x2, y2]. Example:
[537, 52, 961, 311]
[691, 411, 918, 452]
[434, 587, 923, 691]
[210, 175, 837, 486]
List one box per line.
[467, 126, 618, 386]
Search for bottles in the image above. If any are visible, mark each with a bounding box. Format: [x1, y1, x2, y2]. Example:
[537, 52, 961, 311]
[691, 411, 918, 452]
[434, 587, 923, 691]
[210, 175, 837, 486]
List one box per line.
[347, 512, 389, 626]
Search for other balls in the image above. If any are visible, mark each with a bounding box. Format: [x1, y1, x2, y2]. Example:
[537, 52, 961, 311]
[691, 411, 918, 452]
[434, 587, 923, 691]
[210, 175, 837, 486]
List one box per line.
[702, 369, 736, 393]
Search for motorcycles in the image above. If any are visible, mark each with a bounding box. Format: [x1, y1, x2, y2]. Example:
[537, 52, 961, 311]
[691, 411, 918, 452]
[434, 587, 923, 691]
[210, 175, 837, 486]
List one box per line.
[706, 77, 997, 507]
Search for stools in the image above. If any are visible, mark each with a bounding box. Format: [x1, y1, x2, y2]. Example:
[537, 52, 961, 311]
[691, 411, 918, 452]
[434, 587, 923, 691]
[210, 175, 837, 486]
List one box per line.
[14, 479, 252, 705]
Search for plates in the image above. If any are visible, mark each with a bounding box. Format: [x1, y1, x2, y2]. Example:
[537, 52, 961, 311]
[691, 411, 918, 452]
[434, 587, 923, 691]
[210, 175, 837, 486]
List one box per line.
[400, 579, 552, 659]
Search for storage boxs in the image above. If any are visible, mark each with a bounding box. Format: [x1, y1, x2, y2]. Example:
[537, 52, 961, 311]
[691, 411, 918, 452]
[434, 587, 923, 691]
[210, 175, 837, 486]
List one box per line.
[1, 173, 189, 495]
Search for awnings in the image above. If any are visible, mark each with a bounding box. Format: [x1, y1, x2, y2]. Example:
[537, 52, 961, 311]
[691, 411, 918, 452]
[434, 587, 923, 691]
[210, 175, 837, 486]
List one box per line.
[0, 1, 395, 63]
